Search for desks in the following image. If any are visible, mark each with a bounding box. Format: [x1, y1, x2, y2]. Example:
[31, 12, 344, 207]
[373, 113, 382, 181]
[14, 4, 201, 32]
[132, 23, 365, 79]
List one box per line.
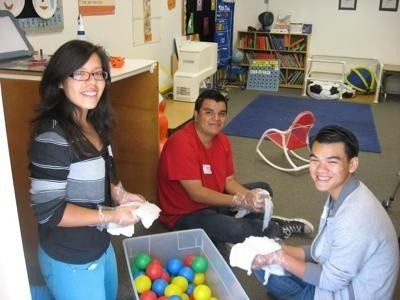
[372, 62, 400, 103]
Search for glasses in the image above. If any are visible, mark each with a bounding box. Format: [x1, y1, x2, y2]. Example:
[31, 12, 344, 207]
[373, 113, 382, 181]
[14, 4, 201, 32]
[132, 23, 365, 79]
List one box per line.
[69, 70, 108, 81]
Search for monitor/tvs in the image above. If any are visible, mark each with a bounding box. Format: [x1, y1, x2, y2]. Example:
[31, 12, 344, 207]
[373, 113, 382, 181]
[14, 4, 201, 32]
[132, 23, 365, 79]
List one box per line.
[0, 9, 34, 61]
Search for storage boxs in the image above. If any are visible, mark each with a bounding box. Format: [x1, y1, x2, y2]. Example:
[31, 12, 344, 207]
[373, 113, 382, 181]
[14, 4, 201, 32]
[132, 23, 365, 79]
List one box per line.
[122, 226, 251, 300]
[172, 41, 218, 103]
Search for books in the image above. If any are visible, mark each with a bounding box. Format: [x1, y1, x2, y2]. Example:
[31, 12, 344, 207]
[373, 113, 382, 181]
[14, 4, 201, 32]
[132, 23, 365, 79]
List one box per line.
[238, 33, 305, 86]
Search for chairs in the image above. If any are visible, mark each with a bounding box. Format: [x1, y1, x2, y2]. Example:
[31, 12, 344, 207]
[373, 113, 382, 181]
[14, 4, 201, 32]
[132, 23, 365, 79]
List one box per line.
[255, 110, 316, 172]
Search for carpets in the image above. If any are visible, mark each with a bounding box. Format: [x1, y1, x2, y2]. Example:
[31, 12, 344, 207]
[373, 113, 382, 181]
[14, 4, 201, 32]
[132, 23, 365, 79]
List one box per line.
[220, 93, 382, 154]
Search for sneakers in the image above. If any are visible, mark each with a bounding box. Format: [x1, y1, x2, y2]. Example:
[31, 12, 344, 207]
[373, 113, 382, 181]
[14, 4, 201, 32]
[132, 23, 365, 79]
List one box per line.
[271, 215, 314, 239]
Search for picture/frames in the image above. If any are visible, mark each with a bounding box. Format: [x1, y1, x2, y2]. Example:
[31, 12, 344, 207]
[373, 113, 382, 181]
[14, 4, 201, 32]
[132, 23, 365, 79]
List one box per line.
[338, 0, 357, 10]
[379, 0, 399, 11]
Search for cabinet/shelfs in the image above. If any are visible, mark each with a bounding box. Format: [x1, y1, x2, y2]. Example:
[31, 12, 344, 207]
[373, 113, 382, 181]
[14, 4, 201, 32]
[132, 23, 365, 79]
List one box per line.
[235, 29, 312, 88]
[0, 53, 161, 288]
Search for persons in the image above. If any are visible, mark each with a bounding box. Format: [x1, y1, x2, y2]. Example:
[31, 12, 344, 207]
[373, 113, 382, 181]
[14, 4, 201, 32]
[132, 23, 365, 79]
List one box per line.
[251, 125, 400, 300]
[156, 90, 314, 243]
[27, 39, 144, 300]
